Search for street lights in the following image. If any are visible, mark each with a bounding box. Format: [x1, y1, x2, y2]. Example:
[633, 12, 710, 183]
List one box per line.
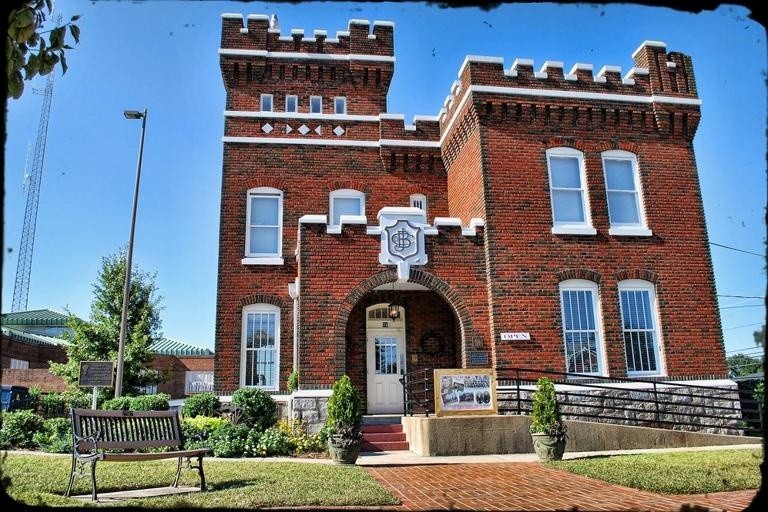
[113, 108, 148, 400]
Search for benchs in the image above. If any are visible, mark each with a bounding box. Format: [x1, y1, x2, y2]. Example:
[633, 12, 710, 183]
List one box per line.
[65, 406, 214, 501]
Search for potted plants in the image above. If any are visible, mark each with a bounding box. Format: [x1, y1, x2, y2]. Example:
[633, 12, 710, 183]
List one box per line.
[324, 374, 363, 464]
[528, 375, 569, 461]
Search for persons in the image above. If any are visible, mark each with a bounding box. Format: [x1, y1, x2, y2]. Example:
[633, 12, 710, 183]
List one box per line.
[441, 377, 451, 392]
